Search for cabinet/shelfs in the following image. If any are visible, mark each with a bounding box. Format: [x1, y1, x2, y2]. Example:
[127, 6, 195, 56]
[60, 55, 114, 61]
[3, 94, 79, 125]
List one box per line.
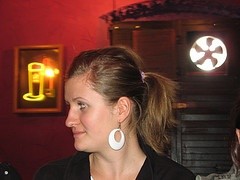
[111, 18, 239, 180]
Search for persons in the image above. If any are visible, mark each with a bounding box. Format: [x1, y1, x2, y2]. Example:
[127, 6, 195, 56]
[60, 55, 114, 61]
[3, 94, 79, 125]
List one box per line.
[33, 45, 197, 180]
[195, 95, 240, 179]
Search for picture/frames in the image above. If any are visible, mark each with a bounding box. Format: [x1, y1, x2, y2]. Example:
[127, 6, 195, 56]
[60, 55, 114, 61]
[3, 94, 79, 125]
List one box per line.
[11, 44, 67, 115]
